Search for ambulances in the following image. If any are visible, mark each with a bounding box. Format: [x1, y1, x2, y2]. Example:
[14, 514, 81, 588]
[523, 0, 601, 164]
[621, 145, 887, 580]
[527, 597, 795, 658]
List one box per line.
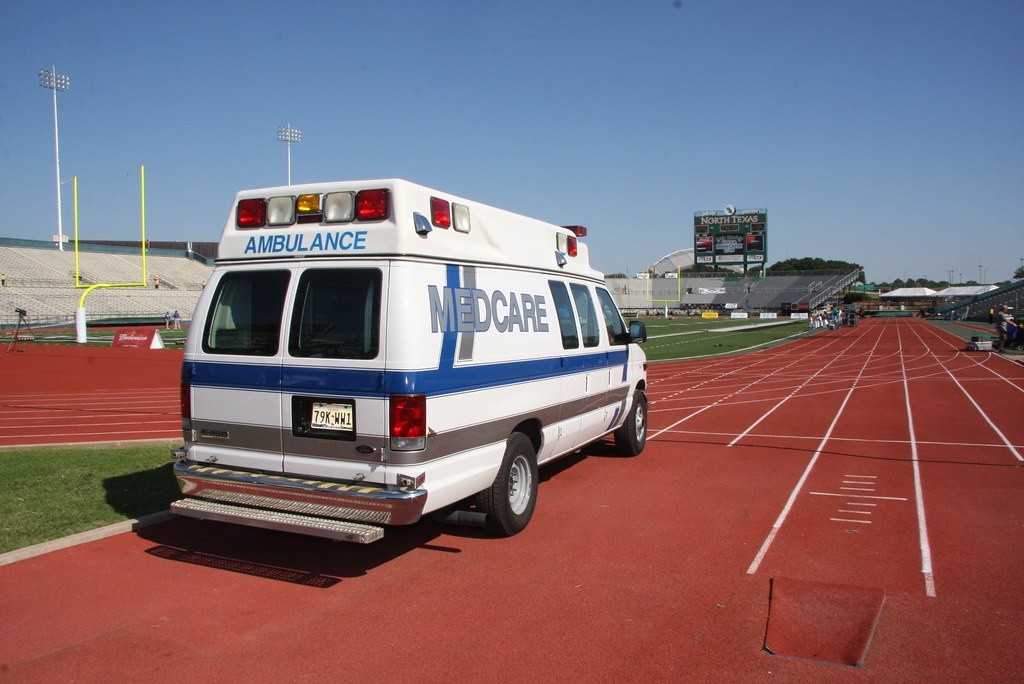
[170, 178, 652, 546]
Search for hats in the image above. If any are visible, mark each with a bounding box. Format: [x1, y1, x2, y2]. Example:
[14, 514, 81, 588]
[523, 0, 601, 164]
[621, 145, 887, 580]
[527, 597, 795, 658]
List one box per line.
[1002, 305, 1007, 309]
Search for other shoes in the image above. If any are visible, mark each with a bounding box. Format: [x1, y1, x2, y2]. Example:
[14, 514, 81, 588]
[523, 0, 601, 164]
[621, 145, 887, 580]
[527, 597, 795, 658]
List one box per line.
[999, 349, 1007, 352]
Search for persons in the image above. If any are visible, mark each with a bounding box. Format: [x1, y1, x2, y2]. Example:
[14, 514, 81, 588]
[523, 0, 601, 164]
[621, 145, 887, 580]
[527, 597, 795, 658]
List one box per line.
[165, 310, 180, 330]
[1, 274, 6, 286]
[997, 305, 1017, 353]
[988, 307, 995, 324]
[815, 305, 847, 331]
[201, 280, 206, 289]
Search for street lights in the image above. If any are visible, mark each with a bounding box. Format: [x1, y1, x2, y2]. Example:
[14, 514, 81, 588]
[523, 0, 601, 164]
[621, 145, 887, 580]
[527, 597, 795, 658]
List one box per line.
[38, 64, 72, 251]
[276, 122, 301, 186]
